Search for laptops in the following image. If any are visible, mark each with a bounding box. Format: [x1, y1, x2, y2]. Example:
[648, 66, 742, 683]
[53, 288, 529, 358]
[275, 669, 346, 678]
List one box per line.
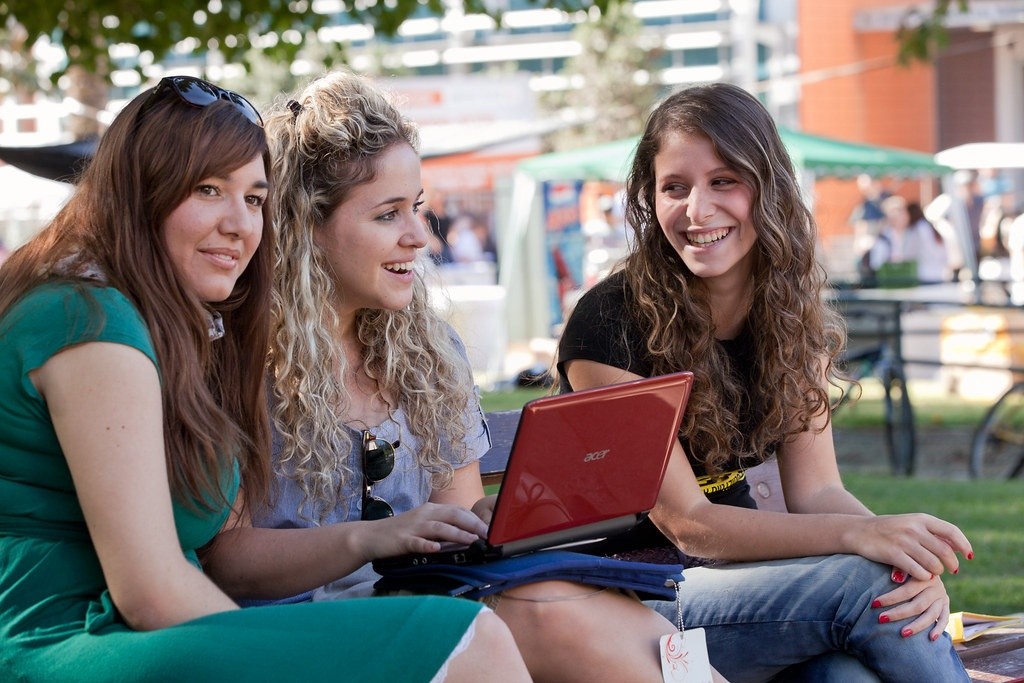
[371, 371, 695, 570]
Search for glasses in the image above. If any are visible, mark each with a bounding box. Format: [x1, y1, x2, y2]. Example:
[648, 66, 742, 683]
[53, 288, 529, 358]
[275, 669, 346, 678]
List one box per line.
[139, 76, 265, 129]
[360, 429, 400, 521]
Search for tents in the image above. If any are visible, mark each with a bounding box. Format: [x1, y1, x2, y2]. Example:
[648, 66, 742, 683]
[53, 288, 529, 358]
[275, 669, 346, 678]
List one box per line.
[490, 124, 973, 346]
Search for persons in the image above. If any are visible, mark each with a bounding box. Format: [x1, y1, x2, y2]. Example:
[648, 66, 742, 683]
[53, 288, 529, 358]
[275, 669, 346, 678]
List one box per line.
[0, 75, 732, 683]
[555, 81, 1024, 683]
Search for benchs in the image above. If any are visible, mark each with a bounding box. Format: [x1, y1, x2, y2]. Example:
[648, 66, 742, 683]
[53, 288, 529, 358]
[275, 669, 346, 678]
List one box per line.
[458, 409, 794, 683]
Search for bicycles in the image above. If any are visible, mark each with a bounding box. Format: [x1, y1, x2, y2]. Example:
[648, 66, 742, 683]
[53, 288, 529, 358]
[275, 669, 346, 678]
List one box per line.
[829, 295, 926, 475]
[969, 363, 1024, 478]
